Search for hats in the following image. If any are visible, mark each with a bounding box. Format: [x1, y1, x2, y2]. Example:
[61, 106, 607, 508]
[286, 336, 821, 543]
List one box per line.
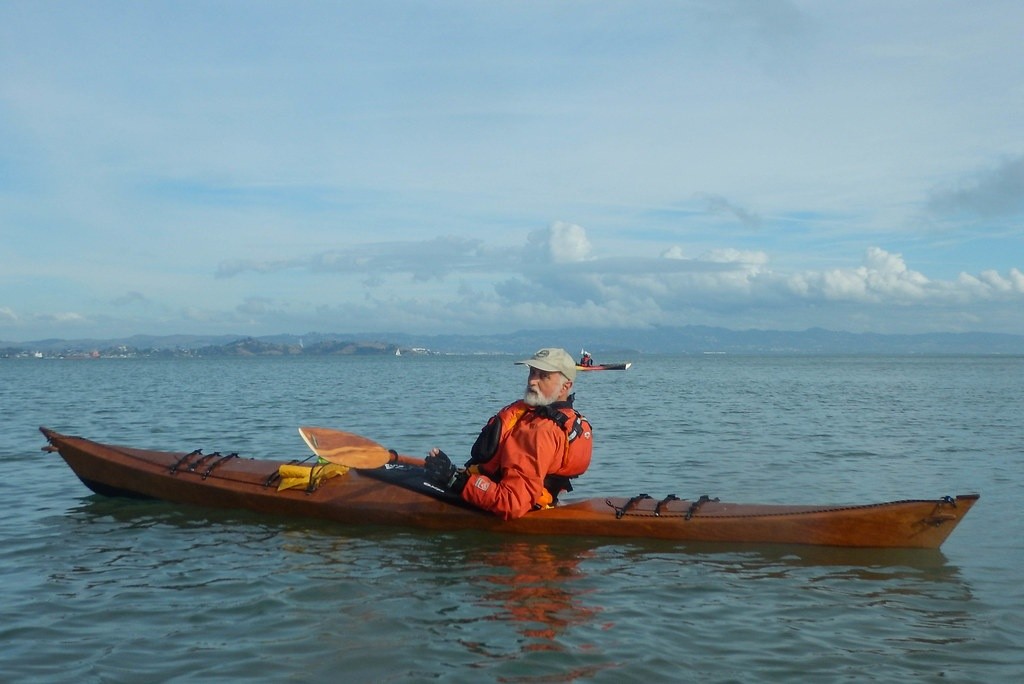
[584, 351, 588, 356]
[514, 348, 576, 382]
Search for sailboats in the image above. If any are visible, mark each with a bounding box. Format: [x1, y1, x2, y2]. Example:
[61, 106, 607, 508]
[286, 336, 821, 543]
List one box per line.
[395, 347, 402, 358]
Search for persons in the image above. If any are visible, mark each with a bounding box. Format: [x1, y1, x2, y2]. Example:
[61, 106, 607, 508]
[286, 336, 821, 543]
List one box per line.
[423, 347, 592, 522]
[581, 351, 594, 366]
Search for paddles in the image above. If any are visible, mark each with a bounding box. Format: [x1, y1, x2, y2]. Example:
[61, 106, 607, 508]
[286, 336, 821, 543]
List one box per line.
[295, 424, 467, 472]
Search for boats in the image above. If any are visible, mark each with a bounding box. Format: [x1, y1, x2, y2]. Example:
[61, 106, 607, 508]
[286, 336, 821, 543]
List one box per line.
[37, 423, 984, 551]
[32, 350, 44, 359]
[576, 364, 633, 371]
